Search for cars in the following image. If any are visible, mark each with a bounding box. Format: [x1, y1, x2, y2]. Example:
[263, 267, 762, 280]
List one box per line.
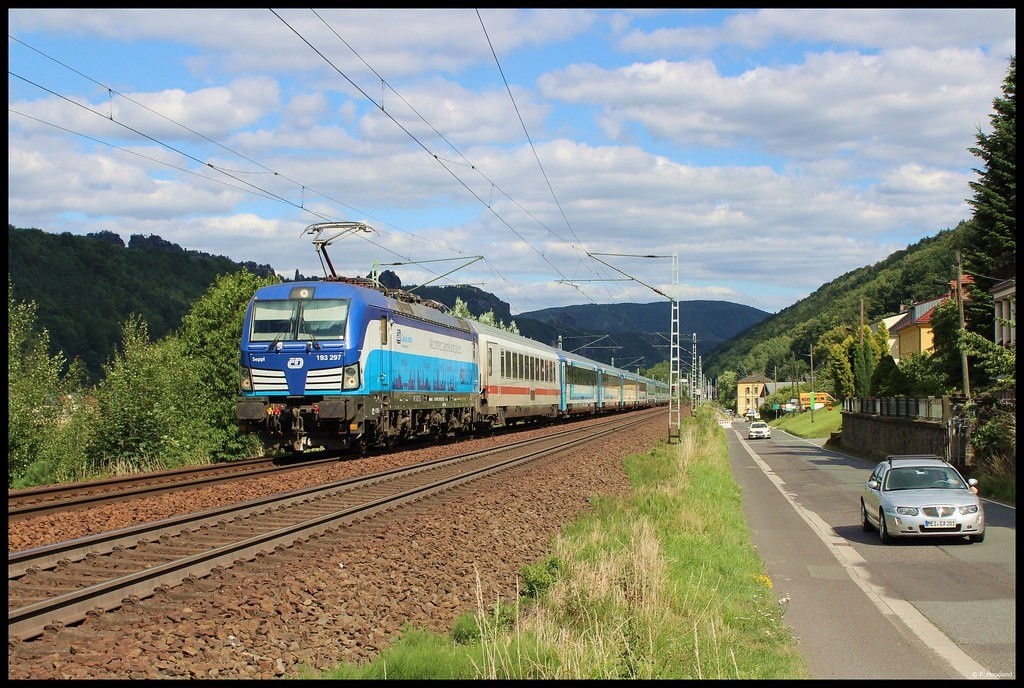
[860, 454, 986, 544]
[748, 421, 771, 440]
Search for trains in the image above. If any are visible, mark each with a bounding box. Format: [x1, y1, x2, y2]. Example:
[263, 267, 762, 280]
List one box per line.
[235, 220, 675, 457]
